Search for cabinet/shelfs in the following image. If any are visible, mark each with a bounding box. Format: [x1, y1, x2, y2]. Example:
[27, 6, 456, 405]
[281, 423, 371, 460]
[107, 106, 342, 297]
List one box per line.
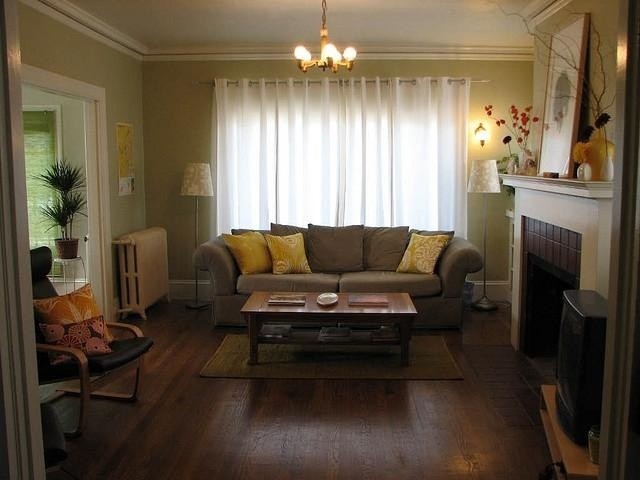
[505, 209, 514, 281]
[539, 383, 599, 479]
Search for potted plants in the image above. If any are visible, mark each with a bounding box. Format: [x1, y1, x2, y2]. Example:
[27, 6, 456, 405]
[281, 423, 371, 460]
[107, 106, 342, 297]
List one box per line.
[26, 159, 88, 258]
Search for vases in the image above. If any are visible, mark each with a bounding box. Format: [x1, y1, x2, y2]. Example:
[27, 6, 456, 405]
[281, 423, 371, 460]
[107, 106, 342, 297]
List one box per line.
[600, 158, 614, 181]
[519, 151, 531, 168]
[507, 157, 516, 173]
[577, 162, 592, 182]
[503, 136, 512, 157]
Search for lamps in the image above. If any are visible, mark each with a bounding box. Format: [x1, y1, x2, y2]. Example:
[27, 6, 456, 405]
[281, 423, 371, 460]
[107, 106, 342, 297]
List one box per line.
[475, 122, 487, 148]
[467, 159, 501, 311]
[179, 162, 215, 310]
[295, 1, 357, 74]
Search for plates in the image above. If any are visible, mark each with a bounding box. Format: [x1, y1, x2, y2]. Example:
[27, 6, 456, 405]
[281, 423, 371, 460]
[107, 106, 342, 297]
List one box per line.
[317, 292, 338, 306]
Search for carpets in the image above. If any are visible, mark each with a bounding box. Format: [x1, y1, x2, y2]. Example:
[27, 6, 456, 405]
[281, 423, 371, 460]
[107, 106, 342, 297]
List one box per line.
[199, 333, 464, 380]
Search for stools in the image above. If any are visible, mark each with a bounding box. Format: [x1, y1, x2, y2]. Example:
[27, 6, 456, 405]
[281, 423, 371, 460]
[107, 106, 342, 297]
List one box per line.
[52, 256, 86, 295]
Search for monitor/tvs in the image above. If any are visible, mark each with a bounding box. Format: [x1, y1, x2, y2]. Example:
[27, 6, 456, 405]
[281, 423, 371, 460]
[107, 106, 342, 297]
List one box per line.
[555, 290, 608, 449]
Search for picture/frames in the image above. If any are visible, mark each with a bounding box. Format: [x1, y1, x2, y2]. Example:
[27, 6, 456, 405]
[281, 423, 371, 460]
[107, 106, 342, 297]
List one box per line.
[536, 13, 592, 179]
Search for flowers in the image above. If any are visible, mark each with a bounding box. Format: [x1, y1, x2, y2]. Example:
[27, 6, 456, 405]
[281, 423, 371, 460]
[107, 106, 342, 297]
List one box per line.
[595, 112, 611, 158]
[484, 104, 540, 151]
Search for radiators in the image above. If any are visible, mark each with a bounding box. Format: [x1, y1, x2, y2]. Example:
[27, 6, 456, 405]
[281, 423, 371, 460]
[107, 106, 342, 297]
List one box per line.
[112, 226, 169, 321]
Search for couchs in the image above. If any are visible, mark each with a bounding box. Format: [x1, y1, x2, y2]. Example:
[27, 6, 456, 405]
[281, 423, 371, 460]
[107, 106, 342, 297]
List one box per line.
[194, 223, 484, 329]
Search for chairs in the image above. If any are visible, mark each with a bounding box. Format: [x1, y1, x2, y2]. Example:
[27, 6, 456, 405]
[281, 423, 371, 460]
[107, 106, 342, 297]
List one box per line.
[30, 246, 155, 440]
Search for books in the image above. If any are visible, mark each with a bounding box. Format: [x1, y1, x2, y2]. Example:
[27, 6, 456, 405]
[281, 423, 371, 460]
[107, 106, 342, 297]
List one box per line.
[267, 294, 306, 306]
[348, 294, 389, 306]
[256, 323, 398, 343]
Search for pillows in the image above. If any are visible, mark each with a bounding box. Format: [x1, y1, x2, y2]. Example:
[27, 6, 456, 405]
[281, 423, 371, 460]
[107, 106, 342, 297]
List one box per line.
[222, 223, 456, 275]
[36, 314, 114, 364]
[33, 282, 119, 345]
